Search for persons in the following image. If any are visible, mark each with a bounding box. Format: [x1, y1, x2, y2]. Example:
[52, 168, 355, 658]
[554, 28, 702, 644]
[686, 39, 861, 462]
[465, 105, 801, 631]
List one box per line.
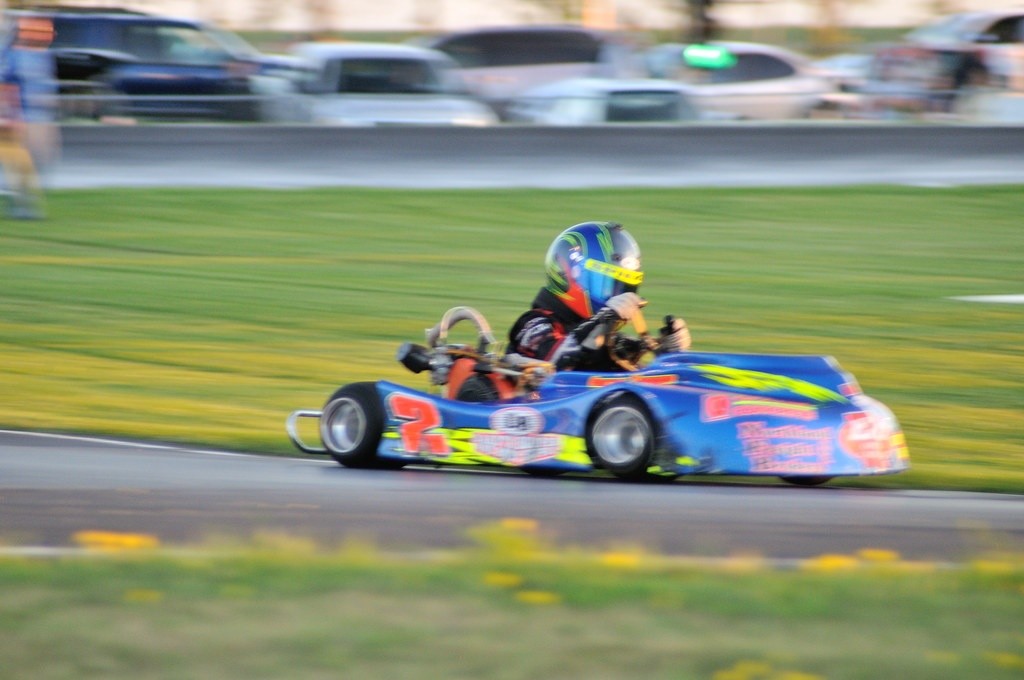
[504, 219, 690, 374]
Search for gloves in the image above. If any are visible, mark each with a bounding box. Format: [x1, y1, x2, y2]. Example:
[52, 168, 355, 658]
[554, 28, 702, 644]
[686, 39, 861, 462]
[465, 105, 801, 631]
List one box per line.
[603, 290, 644, 322]
[655, 314, 691, 357]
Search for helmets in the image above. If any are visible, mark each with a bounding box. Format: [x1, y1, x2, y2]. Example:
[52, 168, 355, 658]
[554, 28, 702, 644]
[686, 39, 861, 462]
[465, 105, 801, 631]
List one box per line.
[542, 220, 644, 320]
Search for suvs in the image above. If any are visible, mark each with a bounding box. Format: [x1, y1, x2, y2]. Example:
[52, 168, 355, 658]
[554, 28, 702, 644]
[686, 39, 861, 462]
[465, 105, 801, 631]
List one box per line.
[429, 25, 604, 92]
[3, 14, 306, 121]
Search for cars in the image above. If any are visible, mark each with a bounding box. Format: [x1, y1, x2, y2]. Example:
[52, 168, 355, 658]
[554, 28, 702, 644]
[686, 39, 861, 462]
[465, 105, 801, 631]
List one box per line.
[613, 44, 853, 122]
[288, 39, 492, 128]
[830, 12, 1022, 118]
